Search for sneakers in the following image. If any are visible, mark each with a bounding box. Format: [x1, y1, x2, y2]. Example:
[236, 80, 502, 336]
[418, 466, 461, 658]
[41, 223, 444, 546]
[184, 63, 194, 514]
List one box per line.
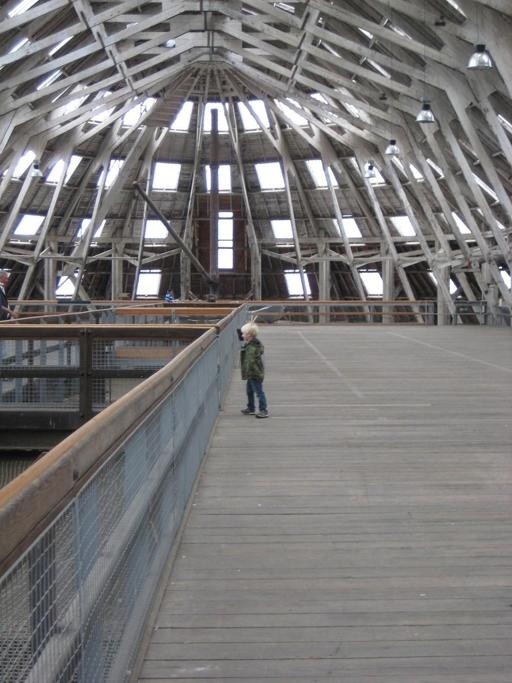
[241, 407, 269, 418]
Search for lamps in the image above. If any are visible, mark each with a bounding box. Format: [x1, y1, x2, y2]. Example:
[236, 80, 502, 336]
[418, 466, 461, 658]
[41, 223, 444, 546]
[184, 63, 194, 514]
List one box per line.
[29, 98, 43, 177]
[360, 0, 494, 178]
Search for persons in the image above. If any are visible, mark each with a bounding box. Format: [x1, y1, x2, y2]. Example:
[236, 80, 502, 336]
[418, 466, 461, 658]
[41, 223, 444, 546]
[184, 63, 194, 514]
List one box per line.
[239, 321, 269, 418]
[0, 269, 19, 358]
[164, 289, 174, 323]
